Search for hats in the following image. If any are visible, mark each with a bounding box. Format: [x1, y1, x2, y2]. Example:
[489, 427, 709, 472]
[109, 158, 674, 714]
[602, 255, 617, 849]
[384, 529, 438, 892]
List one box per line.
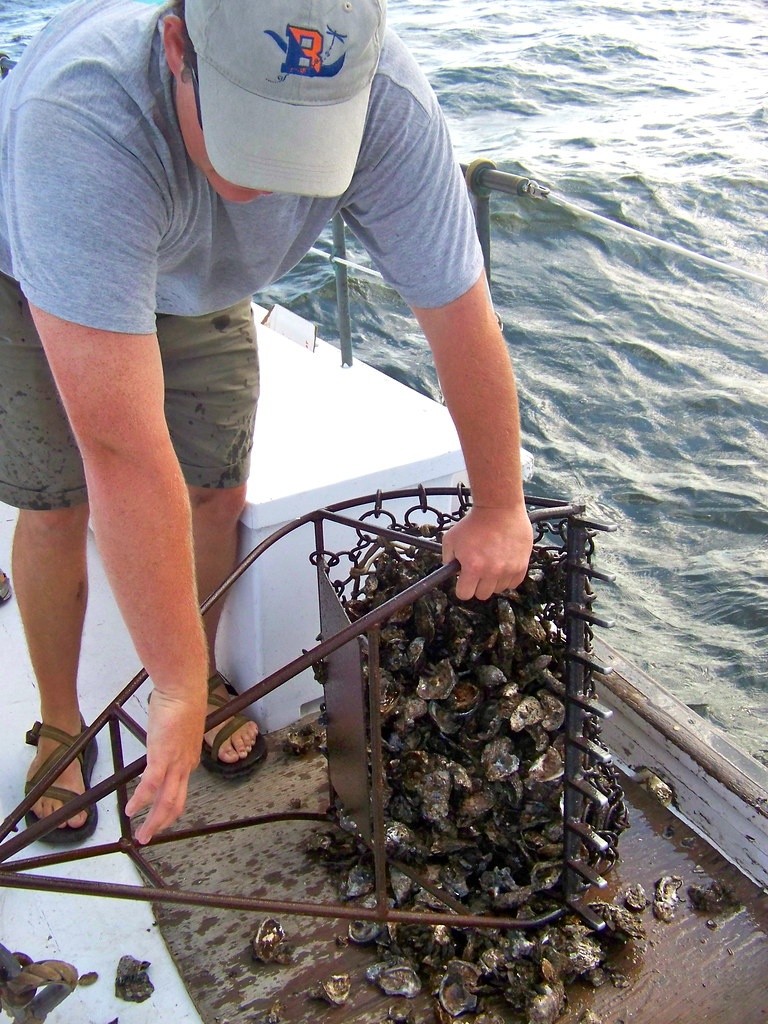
[185, 0, 387, 198]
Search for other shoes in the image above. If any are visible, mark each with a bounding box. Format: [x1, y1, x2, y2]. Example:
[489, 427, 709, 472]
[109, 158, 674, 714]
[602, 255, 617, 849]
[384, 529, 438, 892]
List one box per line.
[0, 568, 12, 603]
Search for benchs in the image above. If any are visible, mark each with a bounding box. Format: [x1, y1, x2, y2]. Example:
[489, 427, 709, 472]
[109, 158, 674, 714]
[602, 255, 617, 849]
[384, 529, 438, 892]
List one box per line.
[88, 159, 549, 737]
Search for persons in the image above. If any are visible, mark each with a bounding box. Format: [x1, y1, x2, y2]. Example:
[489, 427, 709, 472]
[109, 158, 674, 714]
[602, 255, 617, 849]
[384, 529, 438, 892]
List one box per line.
[1, 0, 537, 847]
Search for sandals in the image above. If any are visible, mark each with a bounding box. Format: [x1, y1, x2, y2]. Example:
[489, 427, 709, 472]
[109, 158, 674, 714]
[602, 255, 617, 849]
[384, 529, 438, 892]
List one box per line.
[147, 670, 268, 779]
[25, 709, 97, 844]
